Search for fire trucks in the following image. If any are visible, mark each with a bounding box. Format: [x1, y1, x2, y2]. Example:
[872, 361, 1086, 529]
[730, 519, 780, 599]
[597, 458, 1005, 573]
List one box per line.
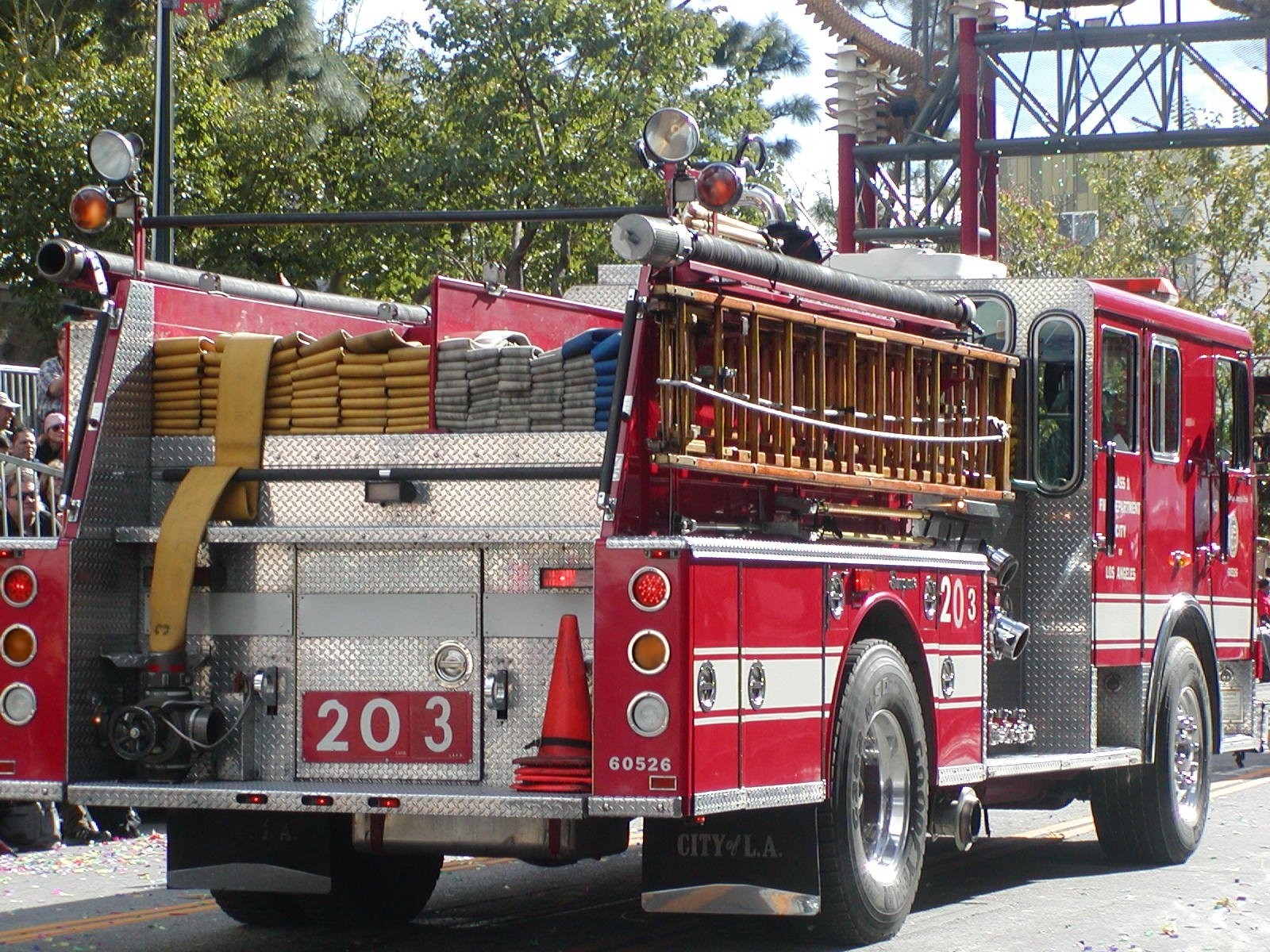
[0, 2, 1270, 938]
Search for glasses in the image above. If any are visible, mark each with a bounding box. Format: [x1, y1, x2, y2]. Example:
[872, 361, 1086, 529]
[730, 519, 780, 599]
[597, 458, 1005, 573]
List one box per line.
[8, 491, 34, 501]
[50, 423, 64, 433]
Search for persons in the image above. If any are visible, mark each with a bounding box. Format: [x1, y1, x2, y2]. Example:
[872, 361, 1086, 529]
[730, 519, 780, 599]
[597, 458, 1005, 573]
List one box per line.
[40, 460, 63, 515]
[0, 472, 62, 538]
[0, 436, 37, 480]
[35, 409, 66, 477]
[0, 801, 142, 854]
[10, 427, 40, 474]
[1257, 565, 1270, 684]
[0, 391, 21, 445]
[36, 328, 66, 433]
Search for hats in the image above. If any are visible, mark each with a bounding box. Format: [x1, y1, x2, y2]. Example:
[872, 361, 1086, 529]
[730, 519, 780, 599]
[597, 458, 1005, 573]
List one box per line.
[0, 392, 22, 408]
[44, 412, 68, 433]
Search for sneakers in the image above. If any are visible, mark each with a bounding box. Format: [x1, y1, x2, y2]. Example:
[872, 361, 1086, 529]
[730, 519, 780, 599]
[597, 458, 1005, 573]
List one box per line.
[64, 828, 113, 846]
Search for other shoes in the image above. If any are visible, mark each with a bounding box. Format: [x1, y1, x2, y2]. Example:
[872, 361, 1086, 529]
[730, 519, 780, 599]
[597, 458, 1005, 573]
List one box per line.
[115, 822, 141, 837]
